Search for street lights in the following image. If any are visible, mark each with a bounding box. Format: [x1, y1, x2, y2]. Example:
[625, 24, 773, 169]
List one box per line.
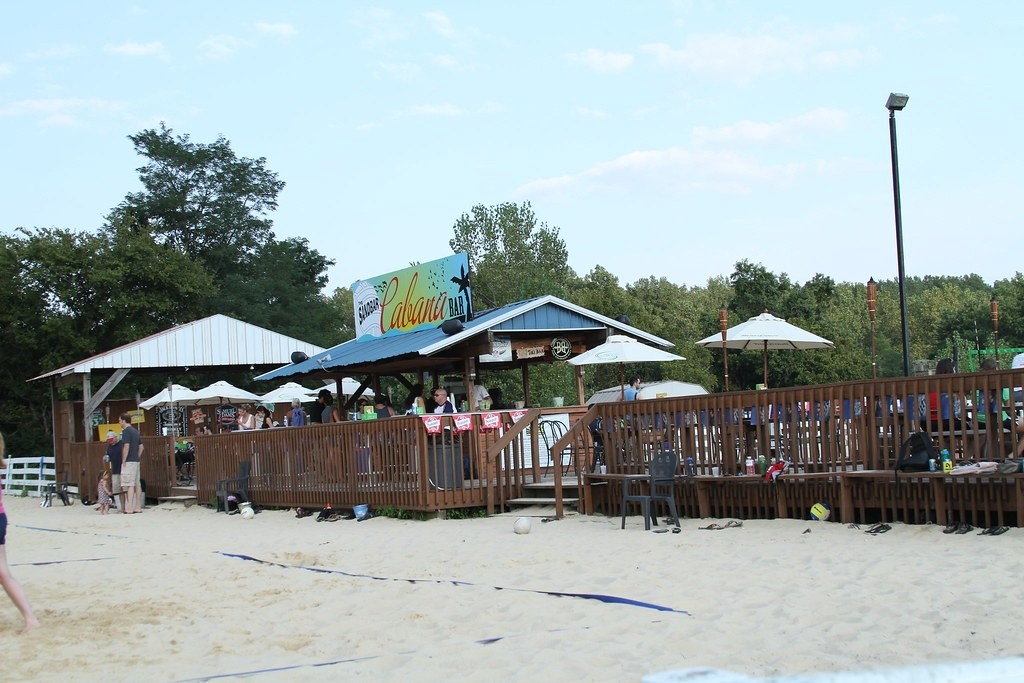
[885, 91, 913, 471]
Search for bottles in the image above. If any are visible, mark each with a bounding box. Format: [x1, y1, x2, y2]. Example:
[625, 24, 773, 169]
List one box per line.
[943, 459, 952, 472]
[686, 457, 694, 475]
[746, 456, 755, 475]
[759, 455, 767, 476]
[941, 449, 949, 472]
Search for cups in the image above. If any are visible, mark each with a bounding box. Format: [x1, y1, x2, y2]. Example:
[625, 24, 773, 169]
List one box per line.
[600, 466, 606, 474]
[712, 467, 718, 476]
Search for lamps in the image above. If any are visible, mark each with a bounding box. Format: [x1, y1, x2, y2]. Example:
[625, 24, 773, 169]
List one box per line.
[316, 354, 337, 373]
[291, 352, 308, 363]
[442, 319, 465, 334]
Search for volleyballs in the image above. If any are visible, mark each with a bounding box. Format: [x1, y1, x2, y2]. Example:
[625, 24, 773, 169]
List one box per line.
[241, 507, 254, 519]
[810, 502, 830, 521]
[65, 497, 74, 505]
[513, 518, 531, 534]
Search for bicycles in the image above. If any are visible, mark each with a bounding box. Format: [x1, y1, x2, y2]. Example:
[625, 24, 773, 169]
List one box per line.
[174, 446, 197, 487]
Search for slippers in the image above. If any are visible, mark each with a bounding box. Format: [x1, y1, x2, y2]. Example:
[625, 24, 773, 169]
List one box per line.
[663, 517, 674, 526]
[991, 526, 1009, 535]
[672, 527, 681, 532]
[698, 523, 724, 530]
[956, 522, 973, 534]
[723, 520, 743, 527]
[943, 521, 958, 533]
[976, 526, 999, 535]
[865, 522, 892, 533]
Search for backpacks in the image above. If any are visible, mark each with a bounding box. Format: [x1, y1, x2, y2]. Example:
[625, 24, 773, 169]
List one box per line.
[895, 432, 936, 472]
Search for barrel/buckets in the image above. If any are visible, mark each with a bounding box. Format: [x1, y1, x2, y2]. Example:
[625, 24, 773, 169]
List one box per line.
[353, 503, 369, 519]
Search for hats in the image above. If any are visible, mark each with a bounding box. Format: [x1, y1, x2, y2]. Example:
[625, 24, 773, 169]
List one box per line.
[107, 432, 116, 442]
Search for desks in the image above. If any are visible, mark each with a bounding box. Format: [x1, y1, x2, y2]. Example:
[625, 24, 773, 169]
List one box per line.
[966, 405, 1024, 412]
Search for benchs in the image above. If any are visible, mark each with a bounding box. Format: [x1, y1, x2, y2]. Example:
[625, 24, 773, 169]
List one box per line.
[879, 427, 1010, 438]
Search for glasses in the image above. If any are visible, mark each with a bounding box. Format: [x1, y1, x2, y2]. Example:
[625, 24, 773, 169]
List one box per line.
[360, 404, 367, 406]
[434, 394, 440, 397]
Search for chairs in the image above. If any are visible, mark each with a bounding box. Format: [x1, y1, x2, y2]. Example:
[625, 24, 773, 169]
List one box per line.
[184, 442, 196, 485]
[216, 461, 250, 514]
[540, 418, 605, 478]
[622, 452, 680, 530]
[46, 470, 74, 507]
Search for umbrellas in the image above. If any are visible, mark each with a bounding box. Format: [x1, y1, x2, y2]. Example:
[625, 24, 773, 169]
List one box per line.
[138, 383, 200, 437]
[693, 308, 836, 387]
[196, 381, 264, 435]
[260, 382, 320, 408]
[563, 334, 688, 402]
[315, 376, 386, 403]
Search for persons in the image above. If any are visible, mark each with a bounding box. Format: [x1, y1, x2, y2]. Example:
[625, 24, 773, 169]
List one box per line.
[234, 405, 274, 430]
[317, 389, 340, 482]
[587, 376, 641, 446]
[287, 397, 307, 426]
[105, 411, 145, 513]
[360, 384, 453, 479]
[919, 349, 1024, 458]
[0, 431, 41, 634]
[467, 378, 493, 409]
[97, 470, 112, 515]
[166, 424, 213, 480]
